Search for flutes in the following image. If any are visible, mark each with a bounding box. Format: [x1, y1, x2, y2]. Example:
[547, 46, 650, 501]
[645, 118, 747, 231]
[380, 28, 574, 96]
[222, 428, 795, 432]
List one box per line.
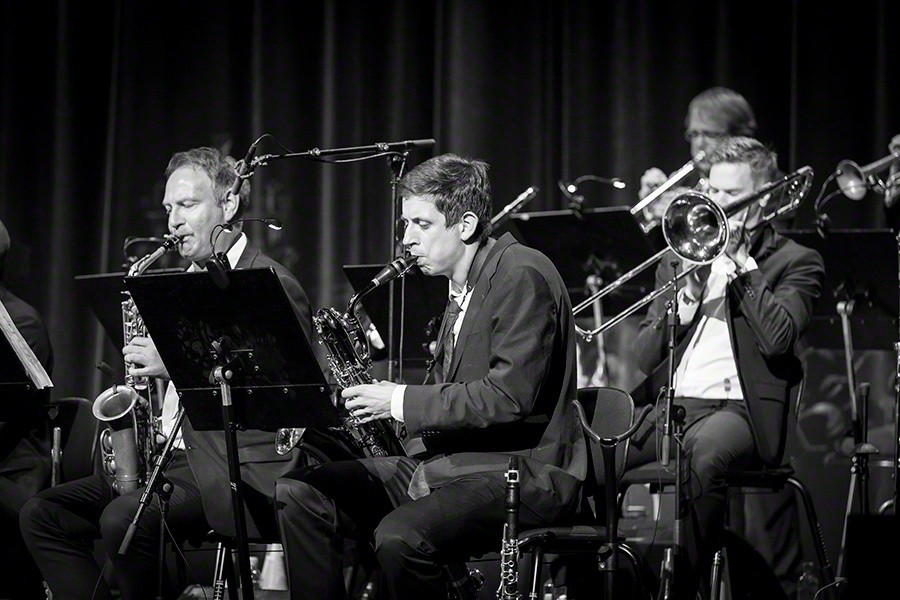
[495, 453, 524, 600]
[42, 426, 65, 599]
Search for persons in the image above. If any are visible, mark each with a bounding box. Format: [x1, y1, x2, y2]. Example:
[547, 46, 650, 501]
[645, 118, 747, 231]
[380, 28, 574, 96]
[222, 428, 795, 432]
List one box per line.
[0, 220, 53, 600]
[19, 147, 314, 600]
[621, 136, 824, 600]
[276, 154, 588, 600]
[638, 86, 797, 242]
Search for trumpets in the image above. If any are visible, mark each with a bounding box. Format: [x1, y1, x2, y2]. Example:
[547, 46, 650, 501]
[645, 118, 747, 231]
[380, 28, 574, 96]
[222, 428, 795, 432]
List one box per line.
[834, 148, 899, 202]
[631, 149, 709, 233]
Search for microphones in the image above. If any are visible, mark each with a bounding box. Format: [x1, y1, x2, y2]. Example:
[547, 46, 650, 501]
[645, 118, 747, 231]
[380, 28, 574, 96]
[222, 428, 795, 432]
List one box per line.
[815, 170, 843, 210]
[231, 143, 256, 195]
[97, 362, 124, 384]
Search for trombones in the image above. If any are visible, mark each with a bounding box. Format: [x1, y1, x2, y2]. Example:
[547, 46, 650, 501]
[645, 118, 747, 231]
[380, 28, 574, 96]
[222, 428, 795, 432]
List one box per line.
[569, 163, 814, 344]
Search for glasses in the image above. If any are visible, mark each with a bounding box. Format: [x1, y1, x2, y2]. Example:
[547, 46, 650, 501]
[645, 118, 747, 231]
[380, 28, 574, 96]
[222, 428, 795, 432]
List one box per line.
[684, 129, 733, 143]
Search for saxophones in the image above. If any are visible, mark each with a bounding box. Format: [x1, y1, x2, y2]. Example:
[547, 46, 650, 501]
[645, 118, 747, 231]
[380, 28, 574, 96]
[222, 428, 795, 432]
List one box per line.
[90, 233, 186, 496]
[274, 254, 420, 464]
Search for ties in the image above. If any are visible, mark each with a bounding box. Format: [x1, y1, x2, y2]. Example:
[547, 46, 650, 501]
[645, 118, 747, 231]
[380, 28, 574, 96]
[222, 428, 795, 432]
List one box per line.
[442, 300, 463, 386]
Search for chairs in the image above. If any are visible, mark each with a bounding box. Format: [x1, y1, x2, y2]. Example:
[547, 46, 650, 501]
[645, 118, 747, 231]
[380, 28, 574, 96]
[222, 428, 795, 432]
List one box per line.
[45, 355, 835, 600]
